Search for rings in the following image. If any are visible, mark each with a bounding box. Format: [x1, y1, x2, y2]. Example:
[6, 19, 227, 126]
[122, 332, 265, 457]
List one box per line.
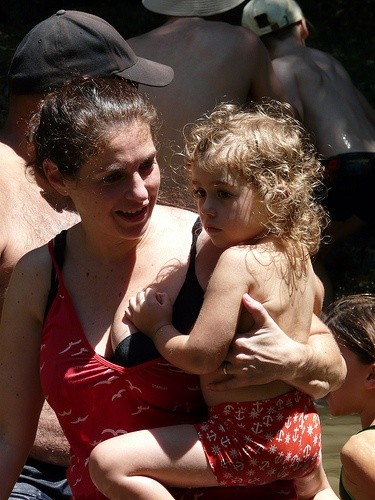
[224, 363, 229, 376]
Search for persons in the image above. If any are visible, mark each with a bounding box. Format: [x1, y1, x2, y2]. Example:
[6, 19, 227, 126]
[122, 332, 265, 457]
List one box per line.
[0, 10, 174, 500]
[239, 0, 375, 307]
[88, 103, 342, 500]
[119, 0, 289, 212]
[0, 75, 347, 500]
[319, 293, 375, 500]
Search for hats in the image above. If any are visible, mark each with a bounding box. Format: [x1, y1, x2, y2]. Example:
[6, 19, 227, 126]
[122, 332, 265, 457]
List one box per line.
[242, 0, 305, 36]
[140, 0, 242, 18]
[7, 10, 174, 94]
